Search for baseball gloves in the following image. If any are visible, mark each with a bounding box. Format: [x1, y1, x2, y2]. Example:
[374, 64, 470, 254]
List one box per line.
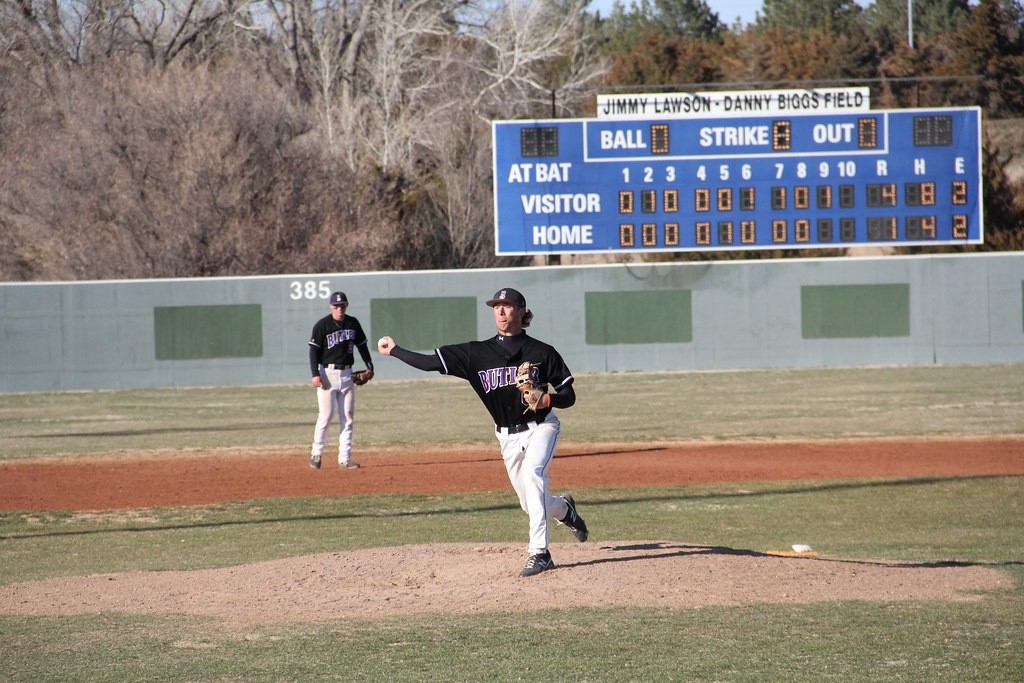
[351, 370, 374, 385]
[516, 361, 545, 414]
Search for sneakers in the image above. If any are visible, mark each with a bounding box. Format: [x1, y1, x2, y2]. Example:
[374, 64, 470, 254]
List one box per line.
[338, 460, 360, 469]
[558, 493, 589, 542]
[310, 455, 321, 469]
[519, 550, 555, 578]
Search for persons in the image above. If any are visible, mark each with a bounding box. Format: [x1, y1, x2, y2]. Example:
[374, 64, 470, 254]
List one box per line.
[309, 291, 374, 469]
[378, 288, 589, 577]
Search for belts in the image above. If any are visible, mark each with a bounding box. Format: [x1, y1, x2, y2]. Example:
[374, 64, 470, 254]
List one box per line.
[497, 417, 546, 434]
[323, 364, 351, 369]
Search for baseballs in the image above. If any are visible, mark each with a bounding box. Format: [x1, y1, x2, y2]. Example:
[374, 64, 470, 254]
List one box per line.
[379, 337, 388, 346]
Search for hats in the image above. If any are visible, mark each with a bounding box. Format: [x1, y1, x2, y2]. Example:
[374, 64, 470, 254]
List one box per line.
[330, 292, 348, 304]
[486, 288, 526, 307]
